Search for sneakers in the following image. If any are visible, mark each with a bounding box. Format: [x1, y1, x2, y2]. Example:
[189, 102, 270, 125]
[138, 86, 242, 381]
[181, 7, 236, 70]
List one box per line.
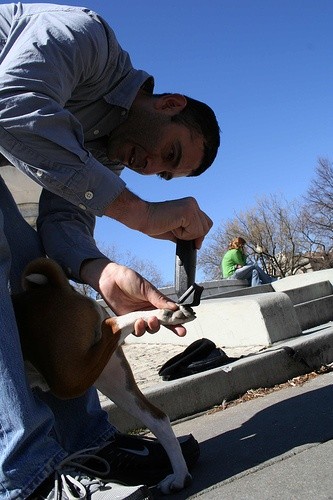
[29, 447, 147, 500]
[81, 433, 202, 479]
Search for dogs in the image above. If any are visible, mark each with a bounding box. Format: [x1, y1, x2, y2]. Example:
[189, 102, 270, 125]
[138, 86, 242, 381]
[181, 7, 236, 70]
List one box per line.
[9, 256, 197, 494]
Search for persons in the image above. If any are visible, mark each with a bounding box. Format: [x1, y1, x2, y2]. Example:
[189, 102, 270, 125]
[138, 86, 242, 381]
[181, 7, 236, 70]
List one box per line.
[220, 236, 279, 288]
[0, 1, 223, 500]
